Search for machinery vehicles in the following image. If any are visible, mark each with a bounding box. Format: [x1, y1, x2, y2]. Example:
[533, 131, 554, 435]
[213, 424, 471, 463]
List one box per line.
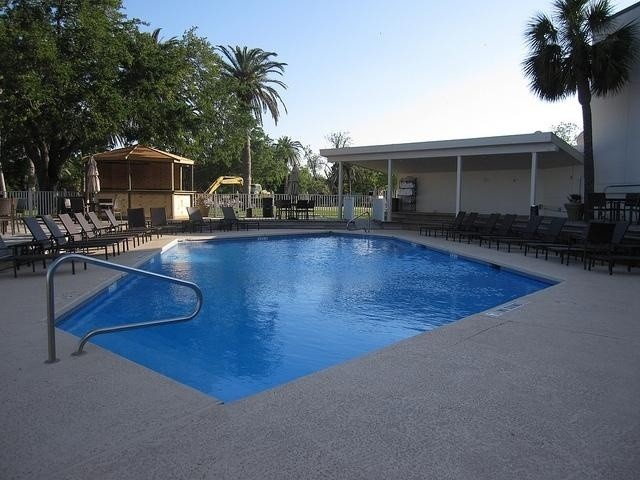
[195, 175, 274, 208]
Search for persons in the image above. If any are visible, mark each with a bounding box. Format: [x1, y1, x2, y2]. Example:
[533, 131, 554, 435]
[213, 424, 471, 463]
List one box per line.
[22, 158, 40, 210]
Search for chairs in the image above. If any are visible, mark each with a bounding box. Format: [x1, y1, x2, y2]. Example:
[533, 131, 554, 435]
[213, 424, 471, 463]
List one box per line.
[418, 191, 639, 276]
[0, 208, 162, 276]
[273, 197, 314, 222]
[124, 206, 261, 232]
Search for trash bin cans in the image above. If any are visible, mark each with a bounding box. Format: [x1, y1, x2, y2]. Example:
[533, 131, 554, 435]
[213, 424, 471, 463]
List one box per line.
[263, 198, 275, 218]
[530, 204, 538, 218]
[391, 198, 399, 212]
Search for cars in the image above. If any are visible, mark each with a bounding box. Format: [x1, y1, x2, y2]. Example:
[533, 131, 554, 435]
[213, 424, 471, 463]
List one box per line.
[195, 197, 246, 209]
[363, 190, 387, 207]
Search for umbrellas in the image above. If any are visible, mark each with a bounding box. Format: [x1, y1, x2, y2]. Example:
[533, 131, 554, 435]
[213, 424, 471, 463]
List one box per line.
[86, 154, 101, 212]
[287, 164, 299, 218]
